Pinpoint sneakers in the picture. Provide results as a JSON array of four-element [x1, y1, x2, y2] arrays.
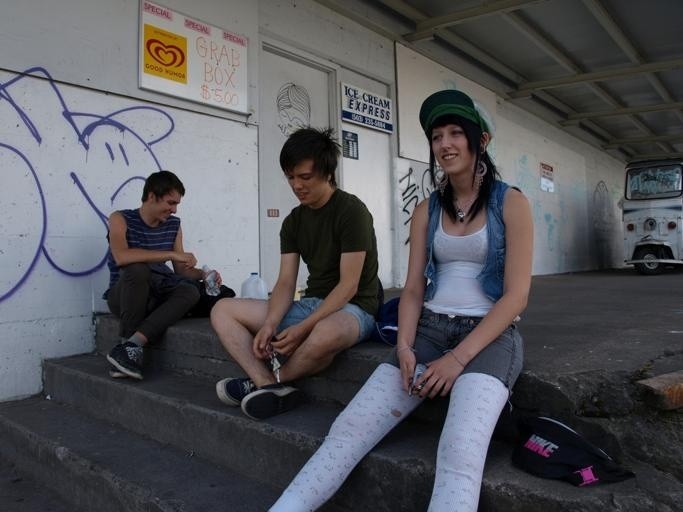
[[106, 342, 145, 380], [239, 383, 303, 422], [215, 376, 259, 408]]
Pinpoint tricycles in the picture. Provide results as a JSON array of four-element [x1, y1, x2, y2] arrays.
[[618, 158, 683, 276]]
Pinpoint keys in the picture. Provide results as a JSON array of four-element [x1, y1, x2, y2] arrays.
[[264, 336, 286, 384]]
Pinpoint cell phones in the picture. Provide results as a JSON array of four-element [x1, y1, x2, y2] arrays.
[[408, 363, 429, 397]]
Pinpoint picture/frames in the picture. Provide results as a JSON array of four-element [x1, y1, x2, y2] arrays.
[[136, 2, 251, 117]]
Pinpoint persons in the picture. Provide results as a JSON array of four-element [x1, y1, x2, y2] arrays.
[[267, 90, 536, 512], [100, 170, 235, 381], [212, 124, 383, 424]]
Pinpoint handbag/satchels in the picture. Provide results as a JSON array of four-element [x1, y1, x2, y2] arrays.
[[511, 413, 638, 487]]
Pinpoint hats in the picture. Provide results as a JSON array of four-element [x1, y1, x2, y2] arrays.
[[371, 294, 400, 347], [418, 88, 495, 140]]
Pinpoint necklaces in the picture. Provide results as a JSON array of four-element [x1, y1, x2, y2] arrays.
[[454, 194, 477, 221]]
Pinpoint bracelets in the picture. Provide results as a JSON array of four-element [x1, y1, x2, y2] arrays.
[[396, 346, 418, 357], [446, 348, 467, 369]]
